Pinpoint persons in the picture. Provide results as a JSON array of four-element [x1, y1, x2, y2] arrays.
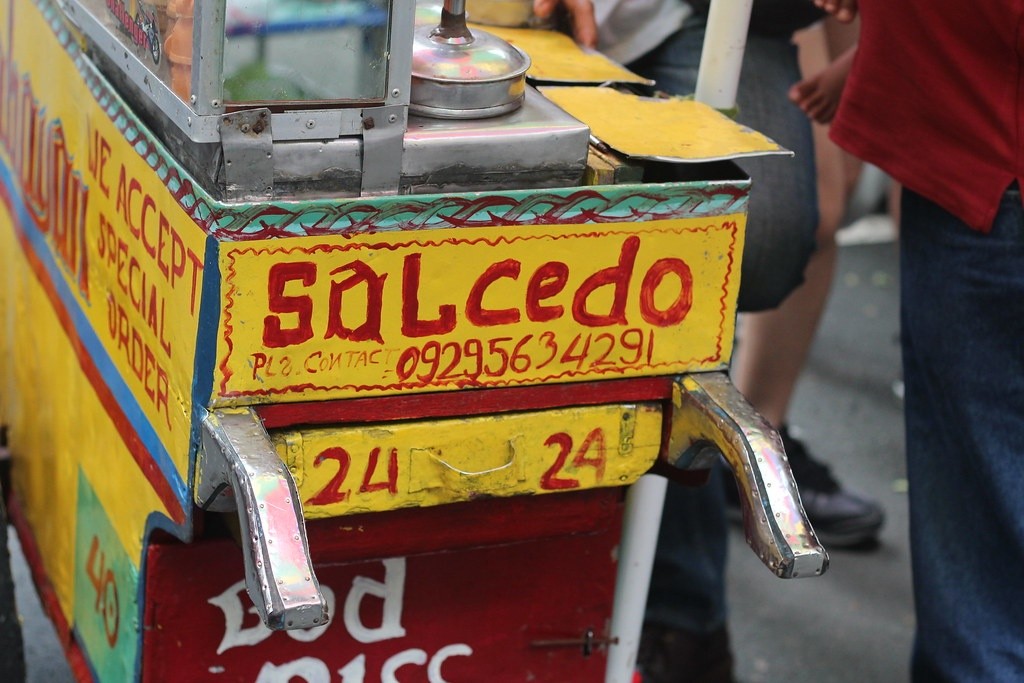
[[786, 0, 856, 123], [832, 0, 1023, 683], [592, 0, 889, 682]]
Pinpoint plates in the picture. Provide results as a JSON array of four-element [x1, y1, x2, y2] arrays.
[[526, 46, 657, 86], [537, 85, 794, 167]]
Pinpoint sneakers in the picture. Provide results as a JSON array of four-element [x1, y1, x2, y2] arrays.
[[724, 425, 887, 546]]
